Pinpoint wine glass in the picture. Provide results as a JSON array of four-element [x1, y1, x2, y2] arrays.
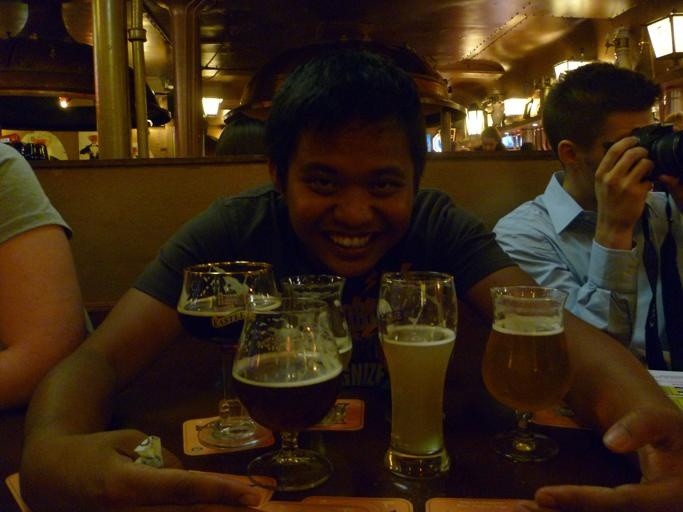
[[484, 284, 567, 463], [231, 297, 343, 494], [176, 259, 283, 451], [287, 275, 353, 452]]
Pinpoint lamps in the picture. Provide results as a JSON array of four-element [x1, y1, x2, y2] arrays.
[[644, 6, 683, 74], [199, 91, 223, 120], [463, 105, 493, 139], [550, 59, 599, 78], [502, 94, 543, 123]]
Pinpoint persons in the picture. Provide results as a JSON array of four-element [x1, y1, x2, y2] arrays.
[[1, 142, 88, 416], [213, 39, 311, 160], [489, 63, 683, 484], [19, 49, 676, 510], [470, 128, 506, 154]]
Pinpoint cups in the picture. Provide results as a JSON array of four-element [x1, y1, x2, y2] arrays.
[[374, 269, 459, 481]]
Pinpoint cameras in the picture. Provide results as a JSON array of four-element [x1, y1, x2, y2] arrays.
[[621, 121, 683, 192]]
